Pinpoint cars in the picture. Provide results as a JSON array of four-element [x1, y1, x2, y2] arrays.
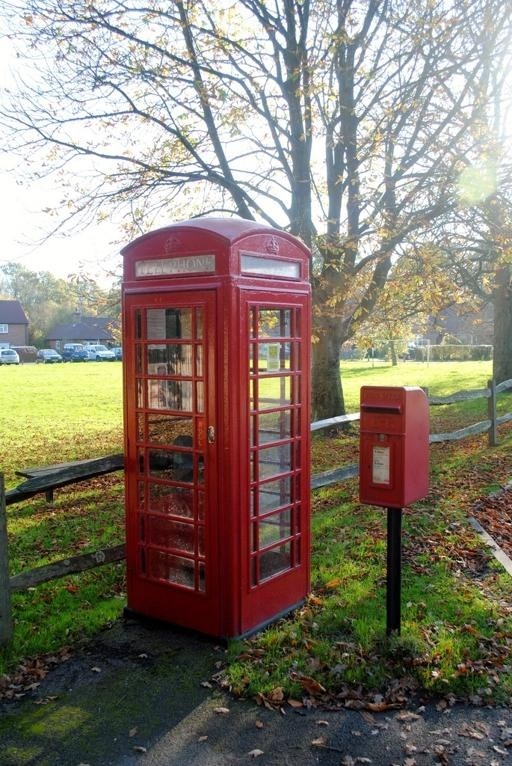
[[111, 347, 123, 360], [1, 349, 20, 366], [36, 350, 62, 363], [263, 344, 290, 359], [63, 351, 86, 362]]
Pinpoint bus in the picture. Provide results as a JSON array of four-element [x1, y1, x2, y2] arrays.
[[64, 343, 84, 352]]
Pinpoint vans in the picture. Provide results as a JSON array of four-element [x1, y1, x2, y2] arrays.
[[12, 345, 38, 361], [87, 345, 116, 361]]
